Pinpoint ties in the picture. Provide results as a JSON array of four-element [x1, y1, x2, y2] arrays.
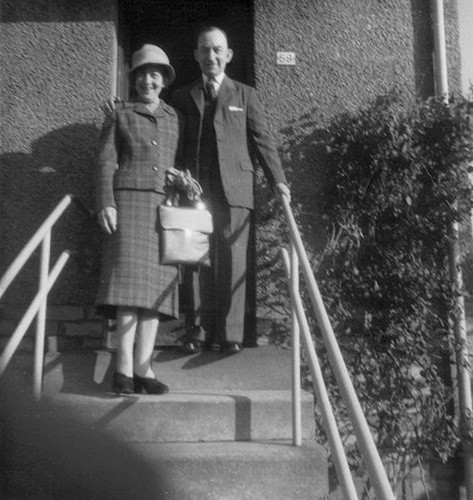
[[204, 79, 217, 100]]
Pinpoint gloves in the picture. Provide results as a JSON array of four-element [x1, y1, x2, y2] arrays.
[[165, 168, 204, 201]]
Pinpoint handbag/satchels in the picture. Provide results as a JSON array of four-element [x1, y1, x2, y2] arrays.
[[156, 186, 214, 268]]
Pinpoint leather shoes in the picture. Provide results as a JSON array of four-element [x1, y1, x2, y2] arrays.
[[221, 342, 240, 354], [184, 338, 207, 355]]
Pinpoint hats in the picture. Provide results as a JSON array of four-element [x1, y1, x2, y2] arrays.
[[125, 44, 177, 89]]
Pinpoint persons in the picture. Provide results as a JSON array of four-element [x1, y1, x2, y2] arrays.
[[98, 24, 292, 355], [93, 44, 204, 395]]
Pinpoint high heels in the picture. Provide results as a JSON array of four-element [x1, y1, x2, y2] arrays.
[[113, 370, 137, 394], [132, 373, 170, 395]]
